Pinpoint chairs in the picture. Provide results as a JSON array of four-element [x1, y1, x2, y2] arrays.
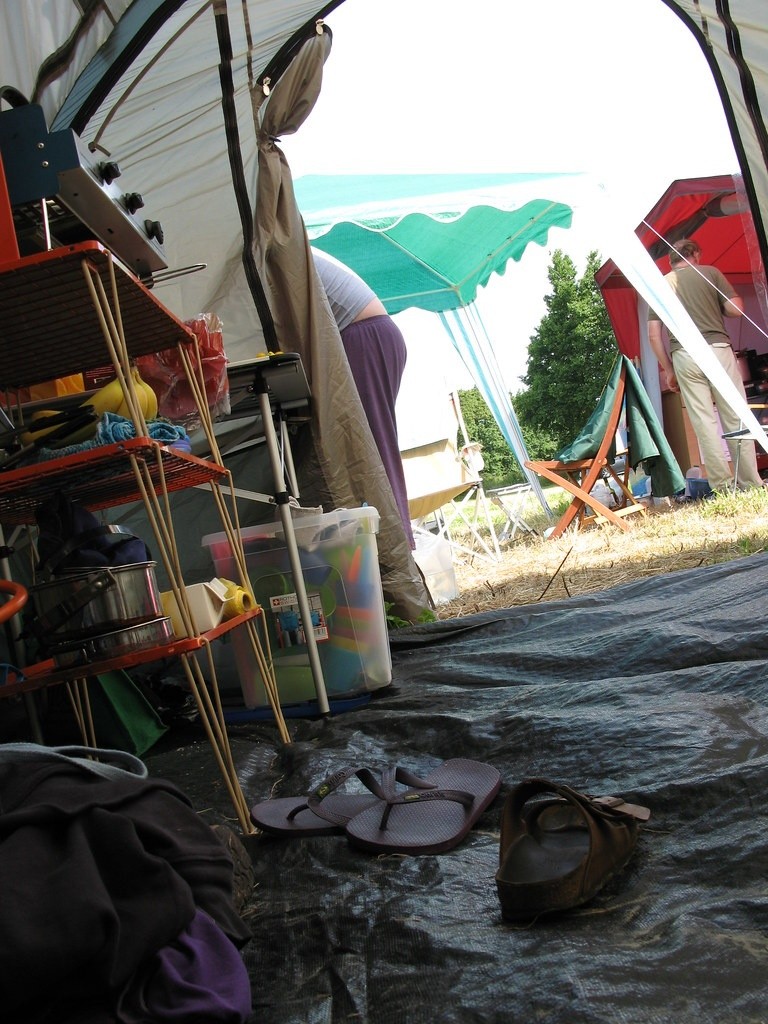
[[722, 403, 768, 497], [525, 355, 649, 541], [399, 439, 498, 561]]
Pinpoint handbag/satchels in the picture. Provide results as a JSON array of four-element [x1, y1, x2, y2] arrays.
[[584, 476, 625, 515]]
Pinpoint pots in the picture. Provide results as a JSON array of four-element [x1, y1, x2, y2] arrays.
[[51, 616, 177, 674], [30, 558, 164, 645]]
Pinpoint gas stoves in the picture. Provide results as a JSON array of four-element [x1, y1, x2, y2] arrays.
[[0, 84, 169, 292]]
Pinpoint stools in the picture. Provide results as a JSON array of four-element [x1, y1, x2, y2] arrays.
[[486, 483, 537, 542]]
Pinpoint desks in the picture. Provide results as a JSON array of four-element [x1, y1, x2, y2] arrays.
[[193, 352, 342, 718]]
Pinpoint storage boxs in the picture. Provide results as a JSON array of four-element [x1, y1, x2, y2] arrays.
[[202, 507, 392, 708]]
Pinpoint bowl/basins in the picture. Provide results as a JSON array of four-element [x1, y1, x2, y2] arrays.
[[688, 477, 710, 499]]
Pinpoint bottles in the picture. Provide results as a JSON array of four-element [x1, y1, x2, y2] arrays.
[[683, 465, 701, 497]]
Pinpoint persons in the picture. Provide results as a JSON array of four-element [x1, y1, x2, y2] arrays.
[[309, 244, 416, 549], [647, 239, 768, 497]]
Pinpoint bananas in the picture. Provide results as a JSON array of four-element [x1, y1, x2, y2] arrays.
[[20, 356, 158, 447]]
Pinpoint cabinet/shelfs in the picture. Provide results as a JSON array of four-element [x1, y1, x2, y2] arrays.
[[0, 152, 291, 833]]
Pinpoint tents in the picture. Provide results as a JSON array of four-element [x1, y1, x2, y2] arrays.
[[283, 172, 581, 520], [593, 173, 768, 358]]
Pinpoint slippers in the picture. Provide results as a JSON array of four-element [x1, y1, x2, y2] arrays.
[[250, 757, 501, 854], [495, 775, 650, 912]]
